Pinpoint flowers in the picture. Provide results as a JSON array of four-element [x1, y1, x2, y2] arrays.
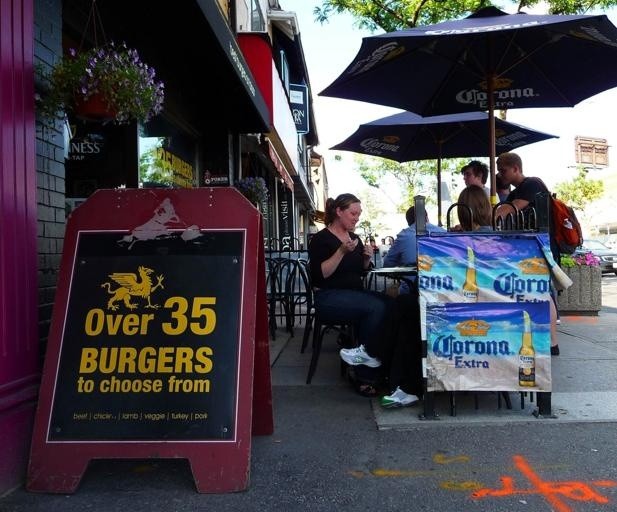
[[234, 177, 270, 202], [42, 41, 166, 124]]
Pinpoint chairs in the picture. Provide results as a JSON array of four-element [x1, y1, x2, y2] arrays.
[[305, 261, 362, 384], [296, 258, 349, 353]]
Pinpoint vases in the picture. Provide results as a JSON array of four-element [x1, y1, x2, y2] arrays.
[[76, 93, 117, 119]]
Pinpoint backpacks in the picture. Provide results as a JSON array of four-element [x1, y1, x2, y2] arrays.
[[549, 194, 584, 254]]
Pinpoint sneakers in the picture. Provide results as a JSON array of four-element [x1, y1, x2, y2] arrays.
[[339, 343, 382, 368], [380, 384, 422, 408]]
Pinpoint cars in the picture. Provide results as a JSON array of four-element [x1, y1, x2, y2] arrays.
[[574, 237, 617, 274]]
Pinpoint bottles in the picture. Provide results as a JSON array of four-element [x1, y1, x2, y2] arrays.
[[462, 262, 479, 302], [518, 326, 535, 386]]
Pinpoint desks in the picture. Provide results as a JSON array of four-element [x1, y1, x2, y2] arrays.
[[367, 267, 418, 340]]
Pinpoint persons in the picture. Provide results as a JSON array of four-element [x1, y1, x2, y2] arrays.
[[311, 195, 420, 408], [457, 153, 498, 228], [382, 205, 446, 296], [494, 152, 560, 356]]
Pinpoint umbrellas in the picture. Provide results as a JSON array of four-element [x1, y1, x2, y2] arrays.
[[318, 5, 617, 232], [329, 110, 559, 227]]
